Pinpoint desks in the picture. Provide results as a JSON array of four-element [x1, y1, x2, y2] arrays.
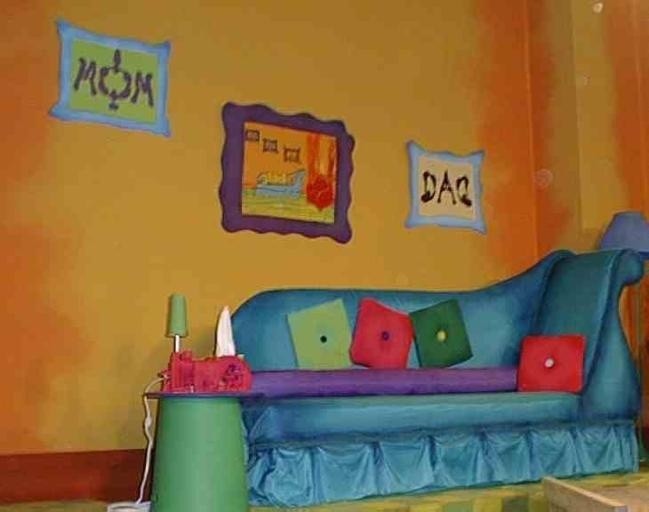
[[144, 391, 259, 512]]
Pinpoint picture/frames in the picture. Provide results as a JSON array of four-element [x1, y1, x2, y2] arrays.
[[47, 16, 172, 138], [218, 102, 354, 244], [405, 141, 486, 235]]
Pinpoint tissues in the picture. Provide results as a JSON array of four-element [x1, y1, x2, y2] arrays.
[[191, 305, 251, 394]]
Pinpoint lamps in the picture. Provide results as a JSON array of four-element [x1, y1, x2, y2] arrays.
[[596, 210, 649, 464], [161, 294, 188, 379]]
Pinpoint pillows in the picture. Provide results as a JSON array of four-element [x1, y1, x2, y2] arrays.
[[517, 334, 585, 394], [349, 300, 413, 368], [409, 300, 474, 368], [287, 300, 354, 372]]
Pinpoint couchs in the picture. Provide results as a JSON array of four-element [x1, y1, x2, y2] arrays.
[[229, 247, 644, 510]]
